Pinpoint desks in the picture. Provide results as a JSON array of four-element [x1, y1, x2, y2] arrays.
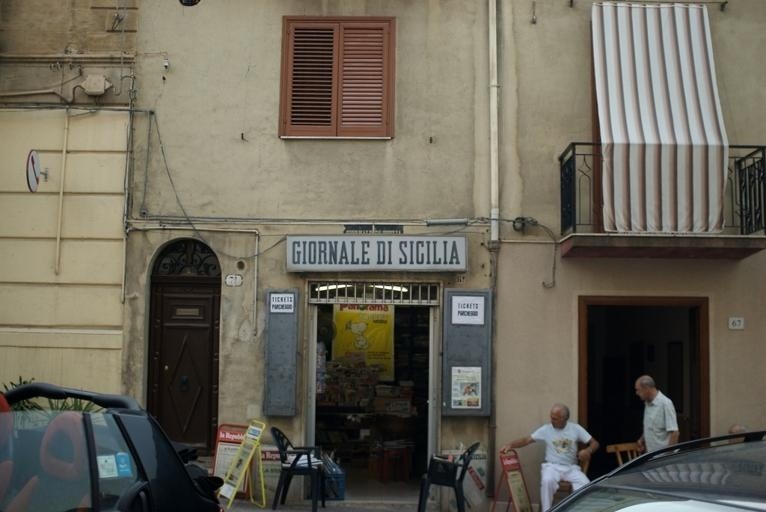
[[316, 404, 424, 480]]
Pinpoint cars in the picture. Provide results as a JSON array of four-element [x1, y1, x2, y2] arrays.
[[544, 430, 765, 511]]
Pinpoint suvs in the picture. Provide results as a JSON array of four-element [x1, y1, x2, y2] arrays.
[[0, 382, 224, 512]]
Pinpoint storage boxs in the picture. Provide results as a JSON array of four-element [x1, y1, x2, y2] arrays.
[[368, 455, 397, 480]]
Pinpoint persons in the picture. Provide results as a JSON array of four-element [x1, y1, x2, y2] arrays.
[[632, 374, 682, 461], [500, 402, 600, 511]]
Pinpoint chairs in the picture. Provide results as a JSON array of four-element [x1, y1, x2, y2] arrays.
[[607, 442, 645, 466], [418, 441, 480, 512], [538, 443, 591, 512], [271, 427, 327, 512]]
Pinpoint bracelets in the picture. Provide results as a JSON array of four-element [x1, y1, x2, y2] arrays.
[[588, 447, 593, 454]]
